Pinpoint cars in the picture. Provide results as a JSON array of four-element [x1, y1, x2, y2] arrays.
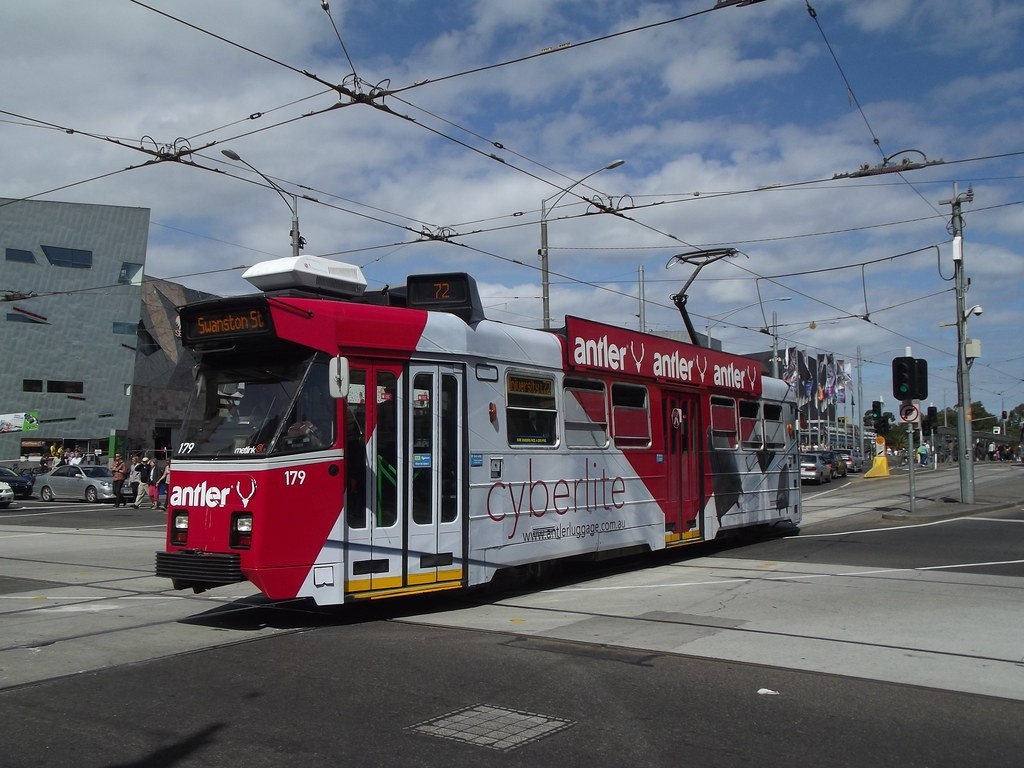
[[805, 451, 847, 480], [832, 449, 863, 473], [31, 464, 134, 504], [0, 481, 14, 509], [0, 465, 33, 500], [799, 453, 832, 485]]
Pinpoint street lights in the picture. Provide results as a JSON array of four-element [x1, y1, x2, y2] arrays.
[[541, 159, 626, 331], [707, 296, 792, 349], [220, 149, 307, 258], [957, 304, 984, 505]]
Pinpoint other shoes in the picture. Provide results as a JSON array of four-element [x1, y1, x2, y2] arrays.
[[152, 505, 158, 510], [131, 504, 138, 509], [113, 504, 119, 508], [124, 499, 128, 505]]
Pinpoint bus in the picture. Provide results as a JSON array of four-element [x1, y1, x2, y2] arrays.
[[156, 247, 803, 609]]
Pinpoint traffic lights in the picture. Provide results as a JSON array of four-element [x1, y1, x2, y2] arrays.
[[872, 401, 880, 420], [874, 417, 886, 436], [1002, 411, 1007, 419], [892, 356, 917, 400]]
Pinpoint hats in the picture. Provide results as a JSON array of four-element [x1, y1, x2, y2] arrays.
[[143, 457, 148, 461]]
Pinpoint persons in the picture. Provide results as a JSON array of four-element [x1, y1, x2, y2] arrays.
[[975, 442, 1013, 462], [918, 440, 930, 467], [225, 382, 290, 417], [111, 452, 128, 508], [40, 442, 85, 467], [894, 447, 908, 462], [13, 463, 22, 475], [886, 446, 892, 456], [128, 456, 170, 511]]
